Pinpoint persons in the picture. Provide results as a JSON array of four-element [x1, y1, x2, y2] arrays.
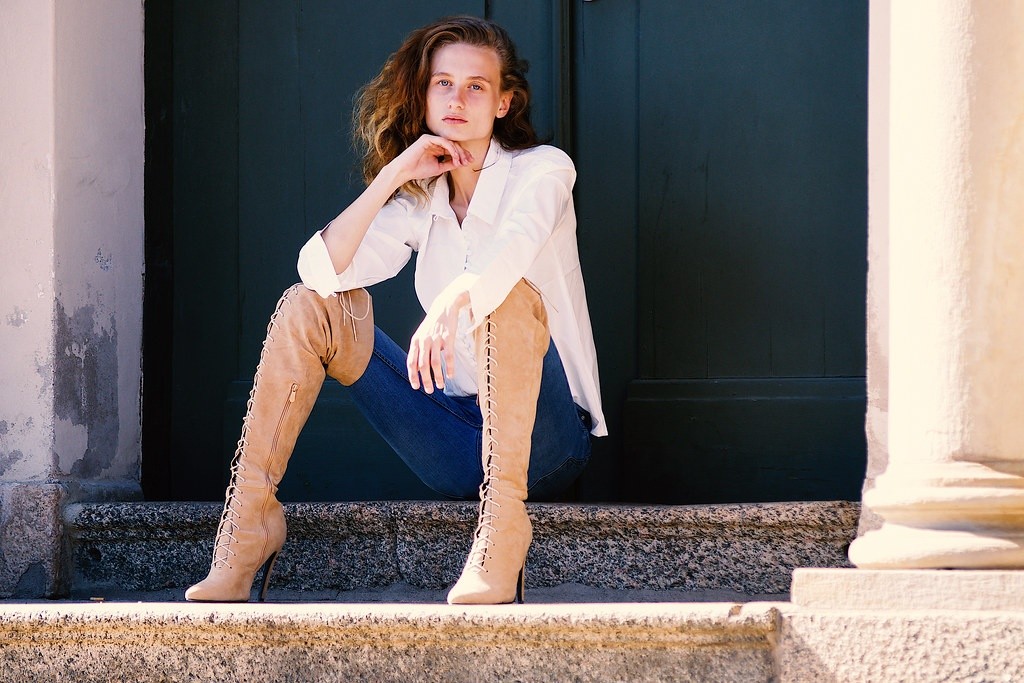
[[183, 13, 606, 605]]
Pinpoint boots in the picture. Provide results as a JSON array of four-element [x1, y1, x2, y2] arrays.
[[186, 283, 377, 602], [448, 278, 552, 606]]
